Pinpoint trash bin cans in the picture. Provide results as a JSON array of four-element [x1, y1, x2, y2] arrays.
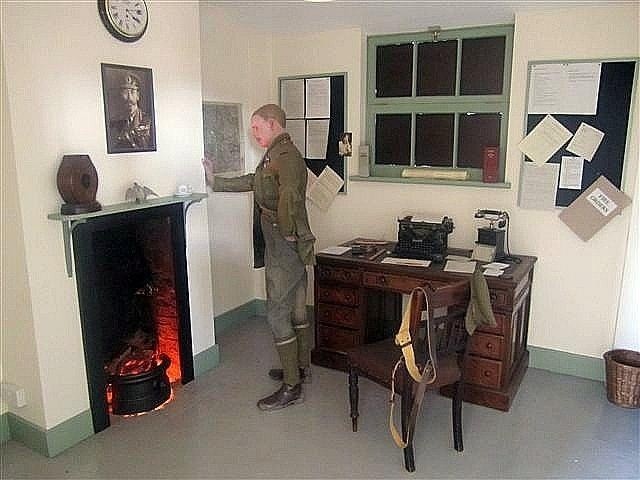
[[603, 349, 640, 409]]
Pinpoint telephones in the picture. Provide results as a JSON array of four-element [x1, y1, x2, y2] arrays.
[[470, 209, 507, 262]]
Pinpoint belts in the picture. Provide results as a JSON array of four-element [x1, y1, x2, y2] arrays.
[[390, 357, 426, 448], [395, 285, 439, 385]]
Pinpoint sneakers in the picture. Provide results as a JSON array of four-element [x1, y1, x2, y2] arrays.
[[258, 384, 304, 410], [269, 366, 312, 384]]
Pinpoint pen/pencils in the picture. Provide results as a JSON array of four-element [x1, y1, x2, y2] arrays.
[[355, 240, 392, 246]]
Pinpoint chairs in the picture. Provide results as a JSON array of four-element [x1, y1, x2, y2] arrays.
[[344, 262, 479, 471]]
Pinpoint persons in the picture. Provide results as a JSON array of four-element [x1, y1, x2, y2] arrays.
[[200, 102, 317, 412], [111, 71, 153, 150]]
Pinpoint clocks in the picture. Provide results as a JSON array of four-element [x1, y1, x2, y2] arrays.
[[96, 0, 149, 43]]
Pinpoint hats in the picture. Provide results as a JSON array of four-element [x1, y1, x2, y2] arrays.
[[120, 71, 142, 90], [465, 270, 497, 336]]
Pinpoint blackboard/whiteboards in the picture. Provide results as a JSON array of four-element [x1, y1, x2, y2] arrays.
[[277, 73, 348, 194], [517, 57, 639, 211]]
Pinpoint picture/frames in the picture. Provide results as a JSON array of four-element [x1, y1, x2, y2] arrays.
[[100, 63, 158, 155]]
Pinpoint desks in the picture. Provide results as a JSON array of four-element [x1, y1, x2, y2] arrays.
[[308, 238, 538, 412]]
